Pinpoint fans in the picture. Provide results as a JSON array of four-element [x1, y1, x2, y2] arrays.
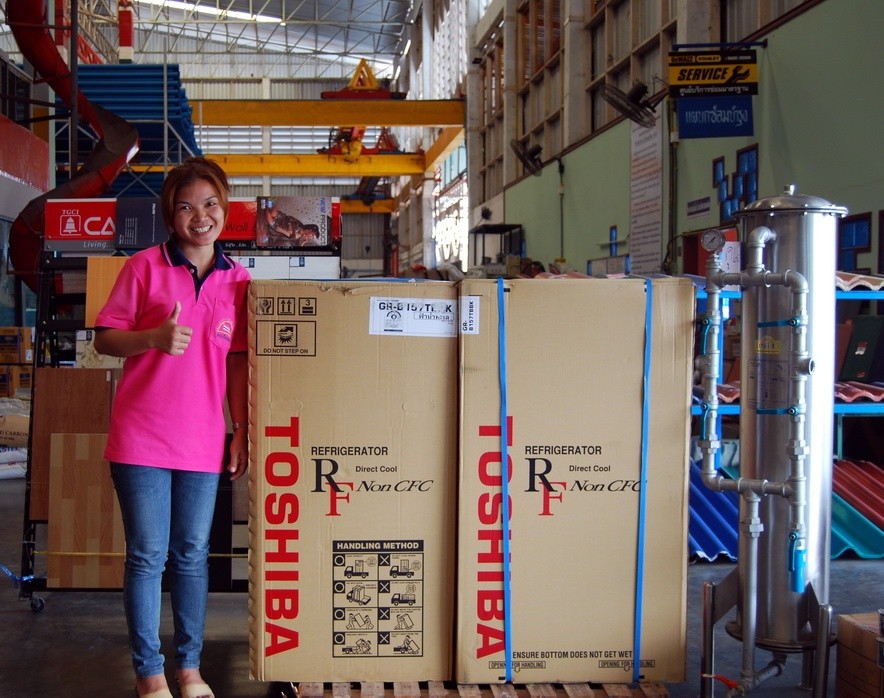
[[511, 139, 543, 176], [598, 79, 671, 130]]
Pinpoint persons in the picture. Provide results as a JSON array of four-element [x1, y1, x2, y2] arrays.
[[257, 197, 320, 247], [92, 156, 252, 694]]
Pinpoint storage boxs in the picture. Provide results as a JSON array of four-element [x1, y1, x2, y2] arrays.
[[455, 280, 696, 686], [245, 279, 460, 684], [0, 327, 36, 447], [834, 612, 884, 698]]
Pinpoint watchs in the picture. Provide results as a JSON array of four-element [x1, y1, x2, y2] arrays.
[[233, 422, 247, 431]]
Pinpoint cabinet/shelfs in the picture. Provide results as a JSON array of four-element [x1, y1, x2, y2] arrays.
[[691, 289, 884, 460]]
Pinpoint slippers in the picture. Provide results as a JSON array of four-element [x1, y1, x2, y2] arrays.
[[134, 679, 173, 698], [174, 674, 215, 698]]
